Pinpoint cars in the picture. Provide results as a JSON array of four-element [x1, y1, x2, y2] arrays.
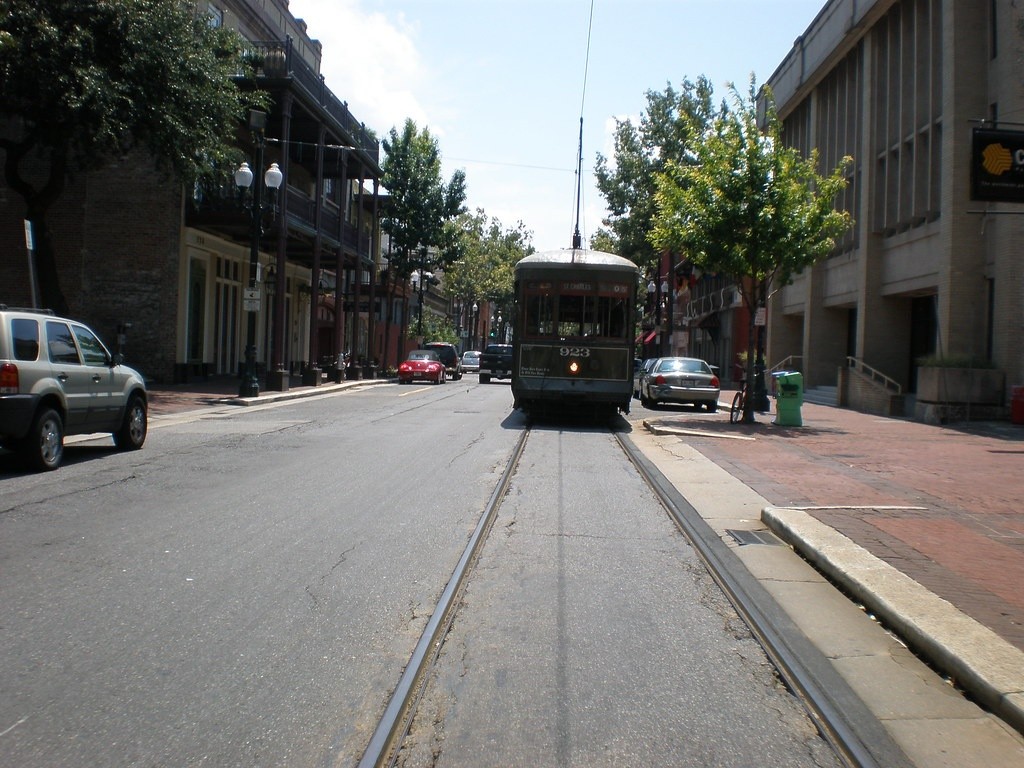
[[632, 358, 659, 401], [458, 350, 483, 374], [398, 350, 446, 386], [640, 356, 720, 413]]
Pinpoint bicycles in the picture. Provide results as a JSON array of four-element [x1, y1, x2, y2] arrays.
[[730, 364, 771, 425]]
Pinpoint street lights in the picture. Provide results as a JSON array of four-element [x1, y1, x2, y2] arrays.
[[411, 269, 434, 349], [468, 302, 478, 350], [235, 127, 283, 397], [647, 280, 656, 358], [490, 315, 503, 344]]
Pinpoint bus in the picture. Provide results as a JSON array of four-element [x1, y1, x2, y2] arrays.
[[509, 247, 643, 418]]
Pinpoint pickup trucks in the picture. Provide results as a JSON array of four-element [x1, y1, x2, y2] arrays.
[[478, 343, 514, 384]]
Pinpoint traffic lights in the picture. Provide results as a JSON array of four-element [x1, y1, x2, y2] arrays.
[[490, 329, 495, 338]]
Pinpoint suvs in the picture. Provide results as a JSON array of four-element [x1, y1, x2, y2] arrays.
[[0, 303, 149, 474], [421, 341, 462, 381]]
